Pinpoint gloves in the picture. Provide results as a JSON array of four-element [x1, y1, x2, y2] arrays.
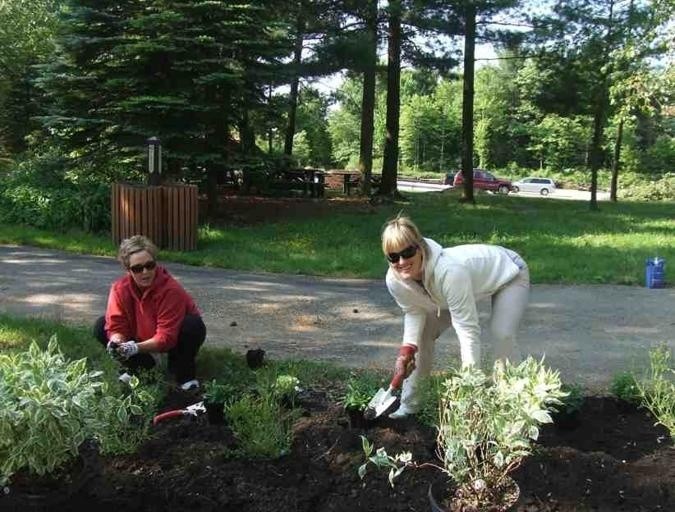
[[393, 343, 419, 378], [105, 338, 138, 368]]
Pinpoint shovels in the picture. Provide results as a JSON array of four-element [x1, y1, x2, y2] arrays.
[[153, 400, 207, 425], [363, 360, 415, 420]]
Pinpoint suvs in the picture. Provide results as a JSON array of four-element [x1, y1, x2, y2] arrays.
[[453, 168, 513, 195]]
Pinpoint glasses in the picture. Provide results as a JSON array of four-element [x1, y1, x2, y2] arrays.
[[126, 260, 156, 273], [386, 245, 419, 263]]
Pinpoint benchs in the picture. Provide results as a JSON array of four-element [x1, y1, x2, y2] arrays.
[[345, 181, 381, 195]]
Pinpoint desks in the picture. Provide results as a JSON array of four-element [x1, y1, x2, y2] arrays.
[[333, 167, 402, 194], [288, 170, 332, 198]]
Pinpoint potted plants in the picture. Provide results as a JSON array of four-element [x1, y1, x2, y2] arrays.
[[357, 351, 572, 512]]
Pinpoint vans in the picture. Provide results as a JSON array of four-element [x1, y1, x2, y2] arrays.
[[511, 176, 557, 197]]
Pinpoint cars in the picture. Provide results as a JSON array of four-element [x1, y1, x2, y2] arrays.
[[444, 169, 459, 186]]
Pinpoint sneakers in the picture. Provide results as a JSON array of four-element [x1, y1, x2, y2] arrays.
[[389, 403, 425, 419], [172, 379, 200, 392], [117, 371, 132, 385]]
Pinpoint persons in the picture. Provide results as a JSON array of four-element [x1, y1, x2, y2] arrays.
[[382, 218, 530, 419], [94, 235, 206, 391]]
